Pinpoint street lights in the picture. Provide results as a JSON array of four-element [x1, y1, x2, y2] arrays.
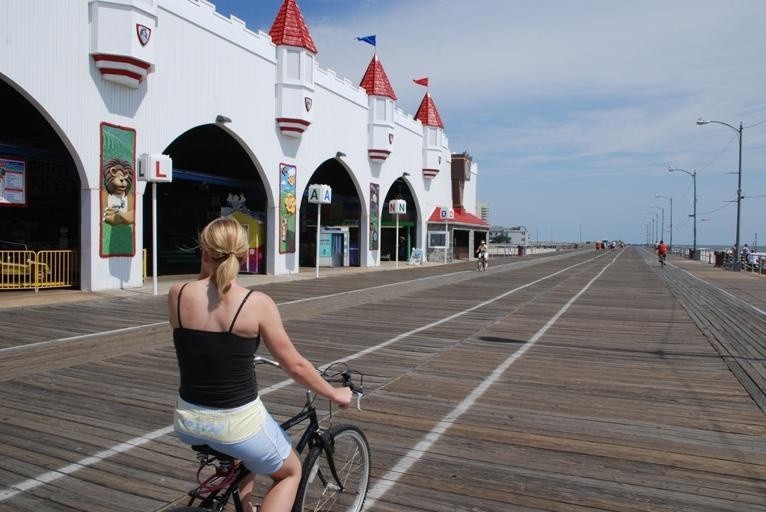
[[644, 193, 672, 252], [668, 166, 698, 260], [696, 118, 743, 271]]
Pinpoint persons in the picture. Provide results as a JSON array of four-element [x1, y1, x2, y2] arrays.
[[733, 244, 737, 267], [658, 240, 667, 264], [166, 215, 353, 512], [475, 240, 488, 269], [748, 249, 758, 268], [743, 244, 750, 263]]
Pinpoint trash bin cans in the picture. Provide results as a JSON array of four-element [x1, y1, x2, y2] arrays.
[[517, 245, 524, 255], [714, 251, 725, 267]]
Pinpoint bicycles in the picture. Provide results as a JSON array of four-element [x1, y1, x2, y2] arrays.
[[659, 255, 666, 268], [476, 250, 488, 271], [166, 353, 372, 510]]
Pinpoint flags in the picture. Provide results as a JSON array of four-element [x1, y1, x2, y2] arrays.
[[356, 35, 376, 47], [414, 77, 428, 87]]
